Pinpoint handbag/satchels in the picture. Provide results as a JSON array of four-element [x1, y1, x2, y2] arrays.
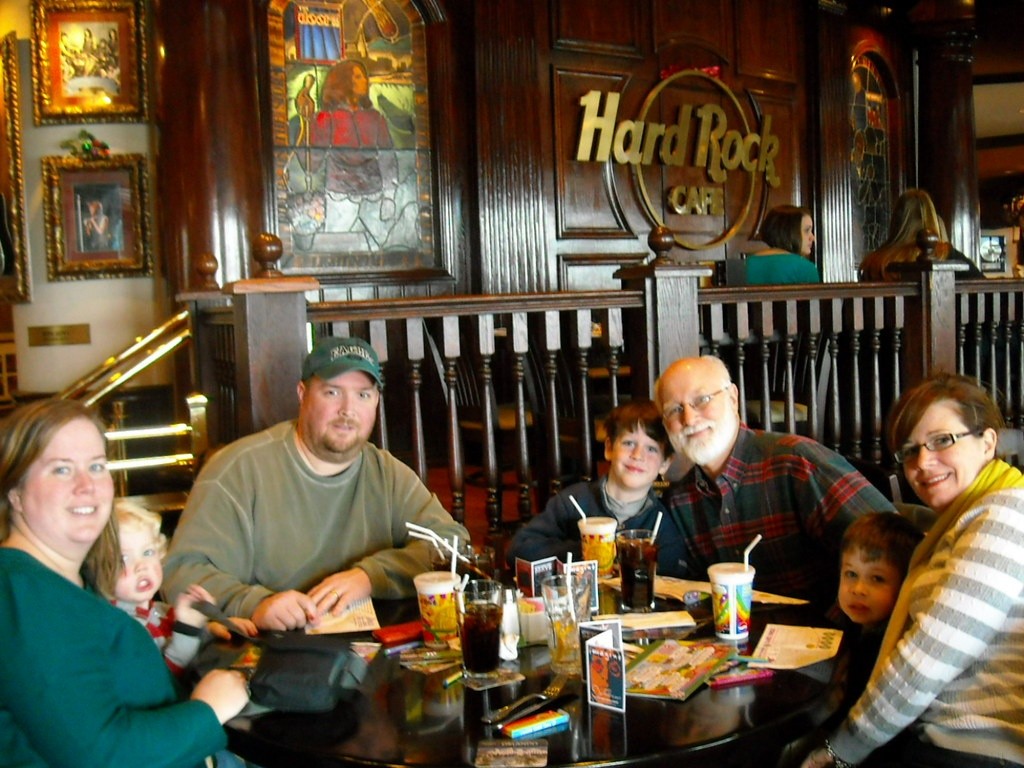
[[170, 601, 365, 711]]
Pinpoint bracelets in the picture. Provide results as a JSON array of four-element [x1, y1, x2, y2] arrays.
[[825, 739, 849, 768]]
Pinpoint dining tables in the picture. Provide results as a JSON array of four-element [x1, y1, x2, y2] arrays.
[[245, 553, 864, 768]]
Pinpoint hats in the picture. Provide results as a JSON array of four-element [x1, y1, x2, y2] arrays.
[[301, 337, 383, 390]]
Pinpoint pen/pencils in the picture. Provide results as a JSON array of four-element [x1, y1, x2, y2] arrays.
[[443, 671, 462, 686], [711, 671, 774, 688], [384, 641, 424, 656]]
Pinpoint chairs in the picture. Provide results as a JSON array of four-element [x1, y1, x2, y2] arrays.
[[560, 312, 648, 425], [498, 313, 637, 478], [420, 312, 535, 491], [745, 324, 827, 437]]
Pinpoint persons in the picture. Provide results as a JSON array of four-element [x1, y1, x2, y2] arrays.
[[0, 398, 265, 768], [505, 398, 686, 575], [160, 337, 473, 634], [857, 188, 986, 282], [798, 371, 1024, 768], [85, 200, 110, 249], [652, 355, 924, 636], [85, 502, 259, 679], [740, 203, 832, 328], [810, 512, 921, 740]]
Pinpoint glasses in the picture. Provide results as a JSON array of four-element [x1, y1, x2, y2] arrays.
[[663, 386, 727, 420], [894, 429, 972, 463]]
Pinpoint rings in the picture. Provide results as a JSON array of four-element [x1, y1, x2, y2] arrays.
[[331, 589, 340, 599]]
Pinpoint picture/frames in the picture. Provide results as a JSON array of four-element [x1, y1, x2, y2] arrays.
[[0, 28, 34, 304], [25, 0, 156, 128], [38, 148, 156, 285]]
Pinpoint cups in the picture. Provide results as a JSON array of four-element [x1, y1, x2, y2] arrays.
[[453, 579, 505, 680], [578, 518, 618, 579], [414, 571, 460, 650], [540, 574, 591, 677], [708, 562, 756, 640], [615, 529, 656, 611]]
